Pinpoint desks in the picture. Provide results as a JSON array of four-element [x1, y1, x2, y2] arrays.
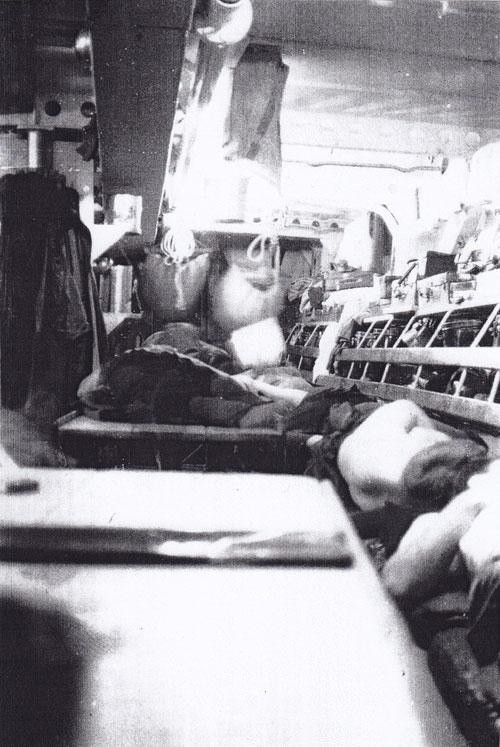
[[0, 468, 468, 747], [51, 411, 308, 473]]
